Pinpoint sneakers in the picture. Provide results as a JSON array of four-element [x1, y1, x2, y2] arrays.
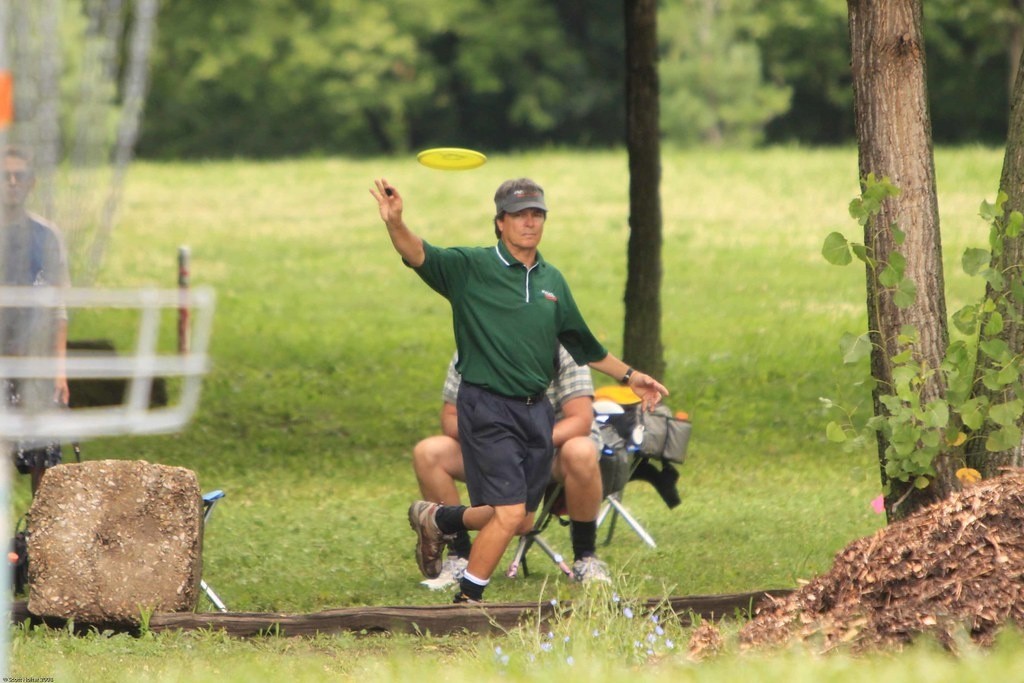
[[572, 556, 612, 587], [419, 557, 469, 590], [452, 592, 481, 604], [408, 500, 457, 579]]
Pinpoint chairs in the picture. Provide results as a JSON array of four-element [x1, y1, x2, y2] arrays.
[[507, 386, 656, 581]]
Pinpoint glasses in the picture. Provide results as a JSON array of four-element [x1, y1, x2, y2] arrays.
[[0, 170, 31, 183]]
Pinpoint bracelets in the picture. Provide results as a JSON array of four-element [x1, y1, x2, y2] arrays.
[[622, 368, 634, 384]]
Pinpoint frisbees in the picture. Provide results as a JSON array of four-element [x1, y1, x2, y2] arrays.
[[417, 147, 487, 171]]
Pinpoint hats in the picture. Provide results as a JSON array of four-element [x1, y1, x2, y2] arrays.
[[494, 177, 549, 215]]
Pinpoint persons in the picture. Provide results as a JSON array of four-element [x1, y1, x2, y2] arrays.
[[414, 342, 610, 592], [0, 145, 69, 474], [369, 177, 669, 604]]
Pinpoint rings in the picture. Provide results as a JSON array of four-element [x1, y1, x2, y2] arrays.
[[385, 189, 392, 196]]
